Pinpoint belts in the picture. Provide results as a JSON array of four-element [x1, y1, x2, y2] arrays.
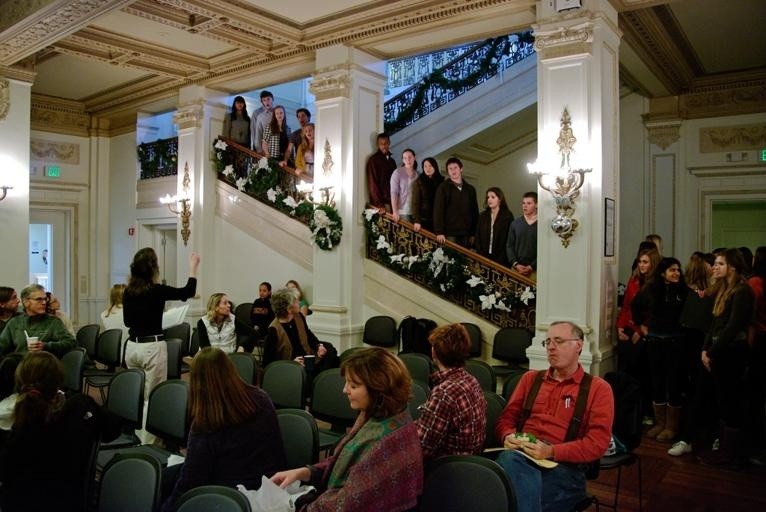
[[129, 336, 164, 343]]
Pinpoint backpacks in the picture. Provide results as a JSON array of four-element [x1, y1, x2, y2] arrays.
[[517, 371, 599, 480]]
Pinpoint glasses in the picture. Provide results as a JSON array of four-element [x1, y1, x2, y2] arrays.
[[541, 338, 578, 347], [25, 297, 48, 303]]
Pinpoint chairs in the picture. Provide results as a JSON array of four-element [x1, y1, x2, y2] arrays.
[[97, 378, 192, 479], [311, 368, 360, 455], [164, 322, 191, 356], [418, 455, 519, 510], [96, 368, 145, 448], [259, 360, 309, 410], [90, 452, 164, 512], [410, 379, 431, 420], [170, 485, 253, 512], [502, 373, 524, 402], [485, 391, 506, 450], [227, 351, 258, 385], [466, 360, 496, 391], [59, 346, 86, 390], [400, 353, 434, 380], [76, 324, 101, 360], [165, 337, 183, 381], [600, 371, 649, 512], [276, 407, 320, 471], [491, 327, 534, 377], [362, 316, 398, 348], [82, 328, 122, 393], [461, 322, 482, 360]]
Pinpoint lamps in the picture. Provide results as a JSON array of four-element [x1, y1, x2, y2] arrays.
[[295, 139, 337, 214], [159, 162, 192, 247], [526, 107, 592, 247]]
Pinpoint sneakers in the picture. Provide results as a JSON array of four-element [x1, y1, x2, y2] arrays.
[[668, 441, 693, 456]]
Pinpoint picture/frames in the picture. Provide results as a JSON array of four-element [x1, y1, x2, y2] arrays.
[[604, 198, 615, 257]]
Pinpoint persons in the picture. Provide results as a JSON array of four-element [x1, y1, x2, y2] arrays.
[[494, 323, 615, 511], [250, 282, 274, 330], [295, 123, 315, 179], [0, 284, 77, 358], [433, 159, 480, 248], [45, 292, 77, 344], [507, 192, 537, 283], [261, 105, 291, 166], [680, 255, 720, 372], [412, 323, 489, 461], [161, 346, 287, 512], [646, 234, 665, 259], [0, 285, 23, 333], [286, 280, 309, 316], [631, 257, 697, 441], [101, 284, 130, 363], [390, 150, 422, 225], [616, 250, 658, 427], [411, 158, 442, 232], [669, 249, 757, 462], [278, 108, 311, 170], [0, 355, 66, 430], [742, 246, 766, 467], [124, 247, 200, 444], [262, 290, 327, 371], [222, 96, 250, 149], [0, 351, 122, 512], [270, 348, 423, 512], [366, 132, 396, 216], [194, 293, 237, 353], [469, 187, 514, 267], [254, 90, 273, 154], [741, 247, 753, 275]]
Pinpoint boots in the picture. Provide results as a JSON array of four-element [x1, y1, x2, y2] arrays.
[[656, 405, 681, 442], [646, 402, 666, 439]]
